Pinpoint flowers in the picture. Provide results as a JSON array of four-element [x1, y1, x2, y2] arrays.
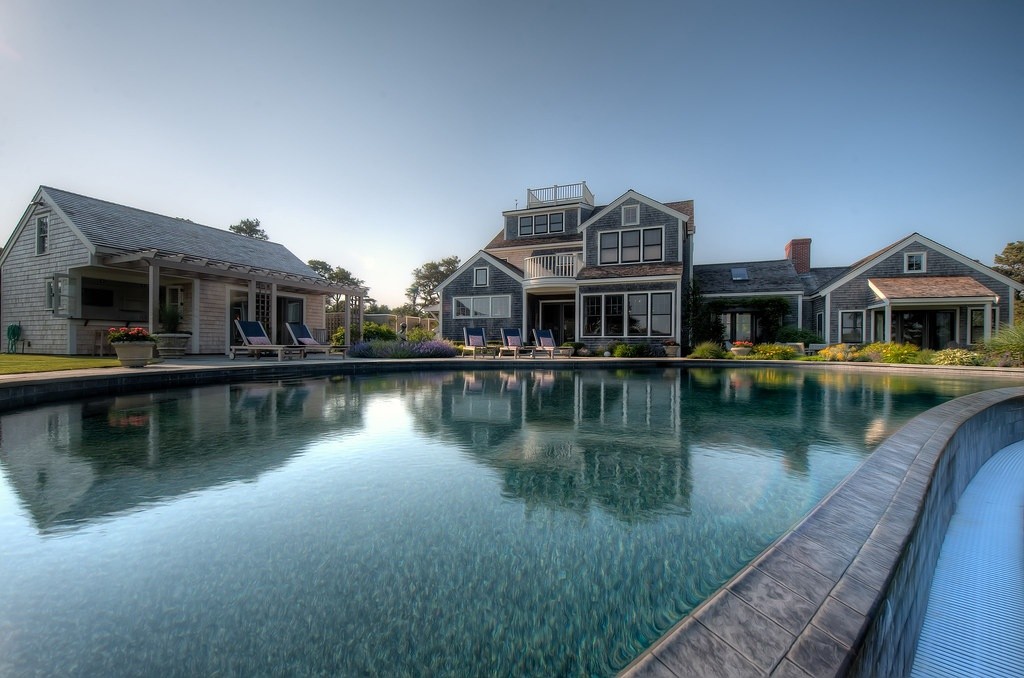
[[661, 338, 679, 346], [733, 340, 753, 348], [106, 327, 155, 342]]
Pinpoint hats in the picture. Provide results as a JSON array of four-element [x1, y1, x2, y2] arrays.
[[400, 322, 407, 327]]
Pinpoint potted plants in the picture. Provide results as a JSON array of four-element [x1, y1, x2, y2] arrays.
[[577, 347, 591, 356], [559, 342, 575, 357], [153, 306, 193, 359]]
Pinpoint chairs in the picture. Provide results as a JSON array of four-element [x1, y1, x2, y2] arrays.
[[462, 327, 497, 360], [531, 329, 573, 359], [284, 322, 351, 360], [498, 327, 536, 359], [230, 320, 306, 361]]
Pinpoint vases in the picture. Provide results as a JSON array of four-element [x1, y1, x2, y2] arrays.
[[730, 347, 752, 356], [663, 346, 680, 357], [110, 341, 156, 367]]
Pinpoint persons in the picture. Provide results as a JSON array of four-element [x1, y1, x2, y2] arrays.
[[398, 322, 407, 342]]
